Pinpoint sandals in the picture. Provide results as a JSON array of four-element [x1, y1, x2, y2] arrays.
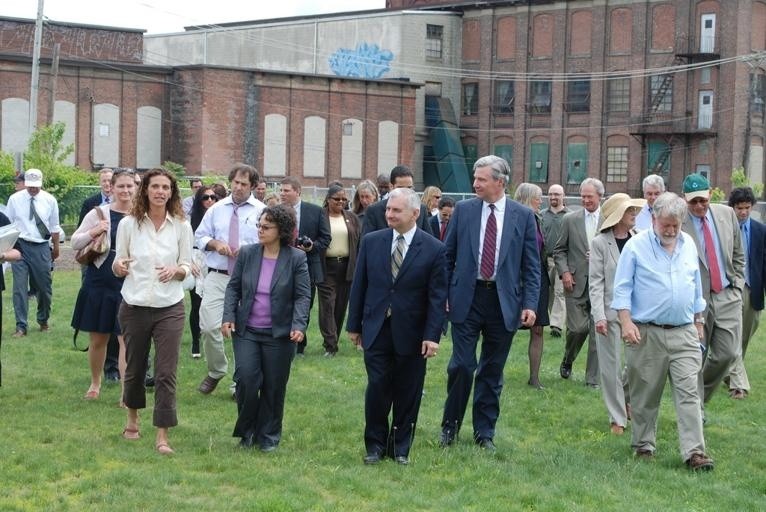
[[154, 444, 175, 454], [84, 392, 100, 400], [105, 375, 118, 384], [191, 340, 201, 359], [121, 428, 141, 442]]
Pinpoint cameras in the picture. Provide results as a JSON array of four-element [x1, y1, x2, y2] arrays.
[[295, 235, 312, 248]]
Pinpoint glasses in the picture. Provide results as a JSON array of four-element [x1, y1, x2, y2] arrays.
[[113, 168, 135, 174], [330, 197, 348, 201], [432, 196, 442, 199], [201, 195, 217, 200]]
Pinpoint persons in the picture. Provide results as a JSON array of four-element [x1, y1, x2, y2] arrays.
[[589, 192, 647, 437], [77, 169, 114, 279], [682, 173, 746, 403], [189, 187, 217, 359], [323, 181, 349, 209], [194, 165, 268, 395], [609, 191, 715, 472], [318, 185, 359, 359], [422, 185, 441, 215], [539, 184, 567, 338], [359, 166, 433, 238], [12, 174, 65, 298], [729, 187, 766, 361], [71, 169, 142, 408], [634, 173, 665, 236], [555, 178, 606, 389], [278, 176, 332, 358], [345, 188, 448, 466], [112, 169, 195, 455], [222, 204, 312, 453], [252, 177, 267, 202], [208, 184, 226, 201], [182, 178, 202, 214], [514, 184, 549, 391], [439, 156, 541, 453], [6, 169, 65, 337], [263, 192, 280, 209], [429, 196, 455, 241], [0, 213, 24, 387], [350, 179, 380, 236]]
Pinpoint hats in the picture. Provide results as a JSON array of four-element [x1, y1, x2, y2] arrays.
[[24, 168, 43, 187], [11, 174, 26, 181], [681, 173, 710, 200], [599, 192, 648, 233]]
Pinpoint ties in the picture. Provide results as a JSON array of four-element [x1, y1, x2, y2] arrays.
[[700, 216, 721, 293], [227, 205, 239, 274], [480, 203, 498, 280], [385, 233, 403, 317], [440, 220, 447, 240], [740, 222, 751, 287], [28, 197, 52, 240], [292, 208, 298, 249]]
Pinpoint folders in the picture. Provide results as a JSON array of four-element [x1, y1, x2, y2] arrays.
[[0, 222, 17, 236]]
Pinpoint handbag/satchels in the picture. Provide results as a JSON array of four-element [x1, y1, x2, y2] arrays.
[[75, 206, 107, 262]]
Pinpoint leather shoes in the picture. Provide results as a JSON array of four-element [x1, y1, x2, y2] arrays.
[[26, 291, 36, 298], [625, 402, 632, 419], [240, 431, 255, 448], [632, 448, 656, 466], [40, 323, 49, 332], [438, 432, 455, 447], [144, 375, 156, 387], [610, 421, 624, 434], [584, 382, 599, 388], [297, 353, 306, 358], [527, 379, 548, 390], [11, 330, 27, 338], [549, 326, 561, 336], [321, 351, 338, 358], [258, 434, 277, 453], [731, 387, 746, 400], [391, 454, 410, 465], [362, 454, 382, 465], [199, 375, 222, 393], [477, 437, 498, 451], [561, 361, 573, 378], [686, 453, 714, 472]]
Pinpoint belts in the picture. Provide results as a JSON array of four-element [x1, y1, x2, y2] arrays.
[[474, 282, 499, 289], [638, 322, 695, 330], [208, 267, 228, 275], [324, 255, 351, 263]]
